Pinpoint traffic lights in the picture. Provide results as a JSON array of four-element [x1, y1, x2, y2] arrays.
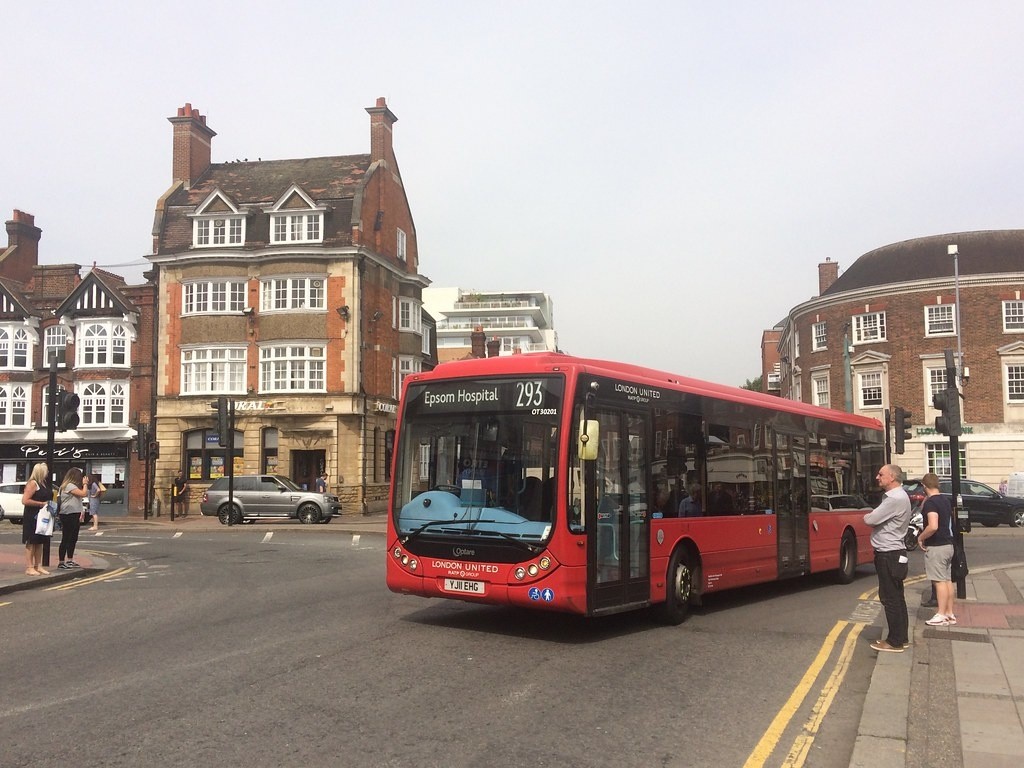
[[894, 408, 913, 454], [210, 397, 229, 448], [149, 442, 158, 460], [931, 387, 961, 437], [57, 392, 79, 432]]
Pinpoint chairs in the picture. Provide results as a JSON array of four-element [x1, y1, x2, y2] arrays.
[[598, 493, 644, 521]]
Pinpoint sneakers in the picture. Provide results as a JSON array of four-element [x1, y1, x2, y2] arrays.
[[925, 613, 957, 626], [57, 564, 73, 570], [66, 561, 80, 567]]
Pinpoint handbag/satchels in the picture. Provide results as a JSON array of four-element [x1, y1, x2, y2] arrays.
[[34, 503, 55, 536], [98, 481, 107, 495]]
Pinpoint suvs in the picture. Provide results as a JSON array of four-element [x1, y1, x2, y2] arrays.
[[902, 476, 1024, 529], [200, 474, 341, 527], [0, 481, 93, 527]]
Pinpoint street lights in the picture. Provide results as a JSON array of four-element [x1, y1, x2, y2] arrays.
[[947, 243, 970, 424]]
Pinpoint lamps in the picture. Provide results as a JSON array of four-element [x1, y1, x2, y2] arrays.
[[241, 306, 254, 315], [336, 305, 349, 320], [369, 311, 383, 322]]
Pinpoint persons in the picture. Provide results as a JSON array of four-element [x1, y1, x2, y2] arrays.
[[999, 479, 1008, 496], [917, 473, 956, 626], [87, 474, 107, 531], [311, 473, 328, 492], [662, 479, 750, 516], [457, 442, 503, 496], [57, 467, 89, 571], [863, 465, 912, 653], [21, 462, 54, 577], [173, 470, 187, 519]]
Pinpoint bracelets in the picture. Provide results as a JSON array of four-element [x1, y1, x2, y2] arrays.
[[83, 483, 88, 485]]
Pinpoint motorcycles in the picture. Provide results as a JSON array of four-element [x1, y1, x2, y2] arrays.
[[904, 503, 928, 552]]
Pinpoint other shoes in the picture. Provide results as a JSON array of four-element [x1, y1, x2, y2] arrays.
[[921, 599, 938, 607], [870, 640, 909, 653], [25, 567, 40, 576], [34, 565, 50, 575]]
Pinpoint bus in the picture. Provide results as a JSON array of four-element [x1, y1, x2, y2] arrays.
[[384, 351, 891, 627]]
